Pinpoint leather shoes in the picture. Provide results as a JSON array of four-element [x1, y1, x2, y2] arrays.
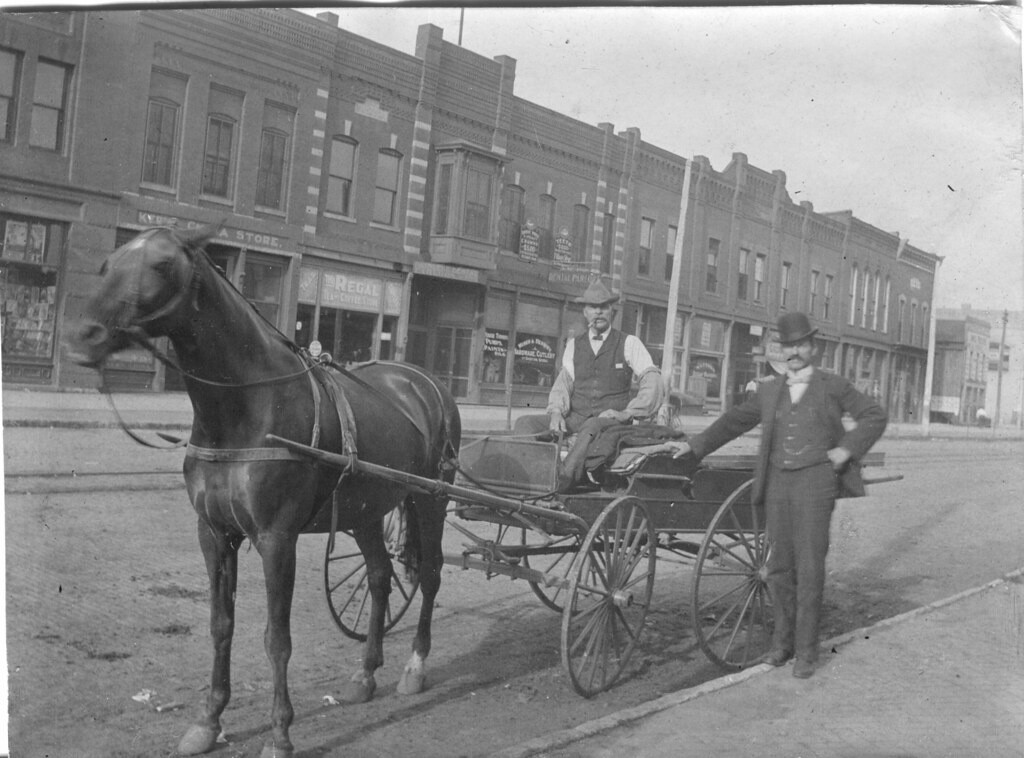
[[792, 652, 815, 678], [762, 646, 794, 666]]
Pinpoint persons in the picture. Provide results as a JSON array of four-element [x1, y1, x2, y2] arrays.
[[665, 313, 889, 682], [512, 281, 664, 499]]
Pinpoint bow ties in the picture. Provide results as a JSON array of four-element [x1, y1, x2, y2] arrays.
[[785, 376, 812, 386]]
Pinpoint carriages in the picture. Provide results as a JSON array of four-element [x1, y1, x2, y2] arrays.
[[61, 218, 774, 758]]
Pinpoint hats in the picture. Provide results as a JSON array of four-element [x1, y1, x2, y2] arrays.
[[771, 312, 819, 345], [574, 280, 620, 306]]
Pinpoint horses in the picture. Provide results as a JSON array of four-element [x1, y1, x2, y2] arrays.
[[63, 216, 462, 758]]
